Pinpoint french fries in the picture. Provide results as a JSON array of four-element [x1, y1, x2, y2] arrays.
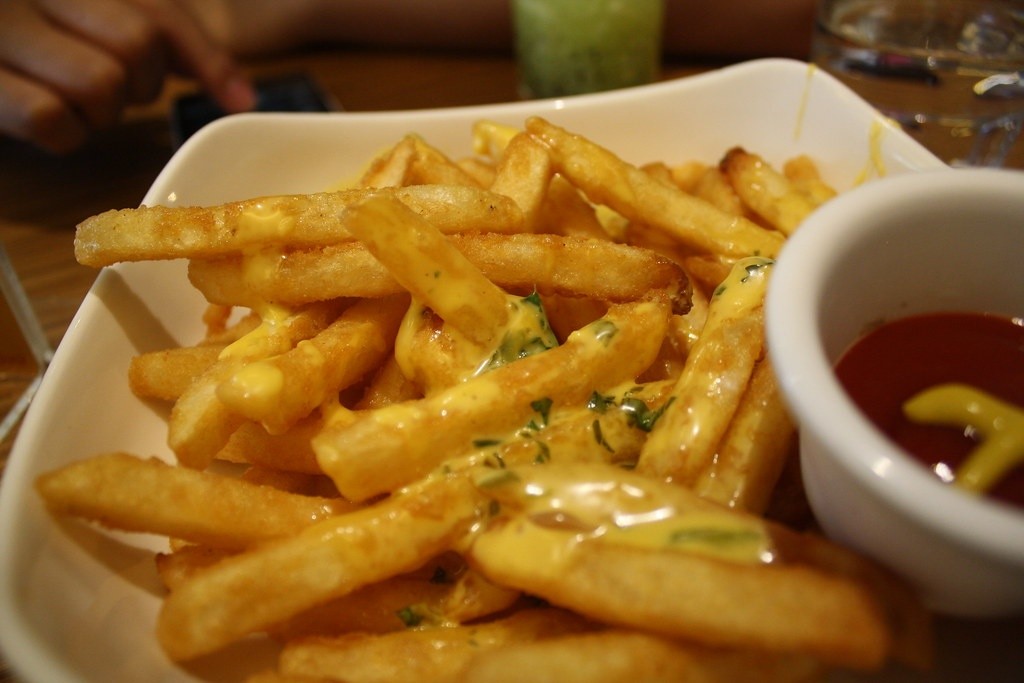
[[32, 119, 935, 683]]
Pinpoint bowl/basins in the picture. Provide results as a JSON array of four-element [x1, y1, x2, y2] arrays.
[[763, 166, 1024, 611]]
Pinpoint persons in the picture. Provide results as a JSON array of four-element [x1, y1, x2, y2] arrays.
[[0, 0, 814, 151]]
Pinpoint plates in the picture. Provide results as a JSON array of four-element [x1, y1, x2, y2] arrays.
[[1, 60, 1024, 683]]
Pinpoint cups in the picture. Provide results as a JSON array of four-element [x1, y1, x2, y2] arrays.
[[514, 0, 663, 101], [815, 1, 1024, 169]]
